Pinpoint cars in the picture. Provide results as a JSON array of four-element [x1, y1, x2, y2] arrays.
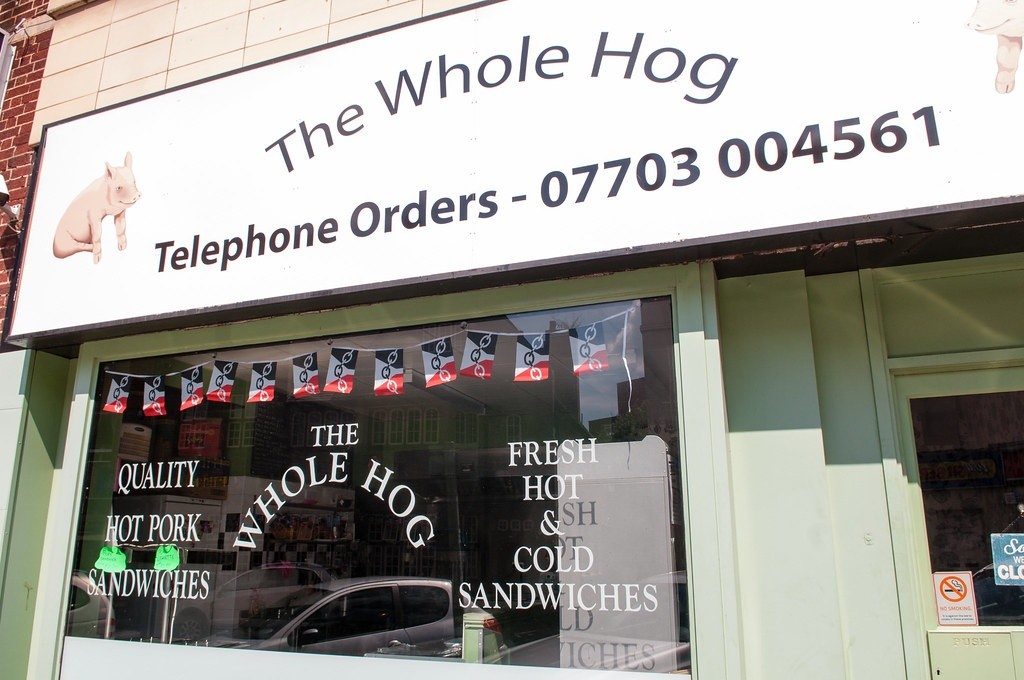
[[67, 569, 116, 640], [178, 573, 512, 666], [612, 570, 690, 643], [170, 559, 344, 645]]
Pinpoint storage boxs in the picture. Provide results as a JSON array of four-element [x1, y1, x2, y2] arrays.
[[112, 495, 222, 549]]
